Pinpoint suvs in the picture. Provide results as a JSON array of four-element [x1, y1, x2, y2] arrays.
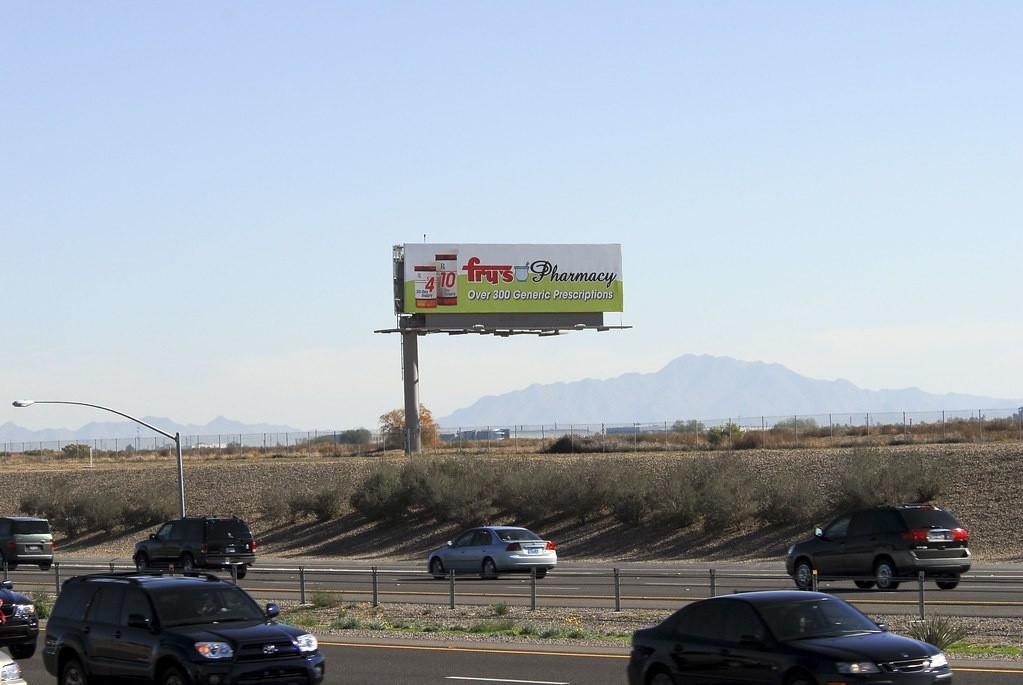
[[42, 572, 325, 685], [784, 503, 972, 593], [132, 515, 257, 579]]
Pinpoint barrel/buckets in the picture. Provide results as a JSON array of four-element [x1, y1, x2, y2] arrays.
[[434, 253, 457, 305], [415, 265, 436, 308]]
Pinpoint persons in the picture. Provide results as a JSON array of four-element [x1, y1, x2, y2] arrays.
[[188, 589, 231, 618]]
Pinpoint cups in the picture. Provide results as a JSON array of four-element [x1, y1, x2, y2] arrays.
[[514, 266, 529, 282]]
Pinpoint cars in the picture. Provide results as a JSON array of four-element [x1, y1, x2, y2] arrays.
[[0, 580, 39, 660], [427, 526, 560, 582], [0, 516, 54, 573], [626, 591, 954, 685]]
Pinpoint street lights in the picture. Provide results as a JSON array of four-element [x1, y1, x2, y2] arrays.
[[12, 399, 186, 519]]
